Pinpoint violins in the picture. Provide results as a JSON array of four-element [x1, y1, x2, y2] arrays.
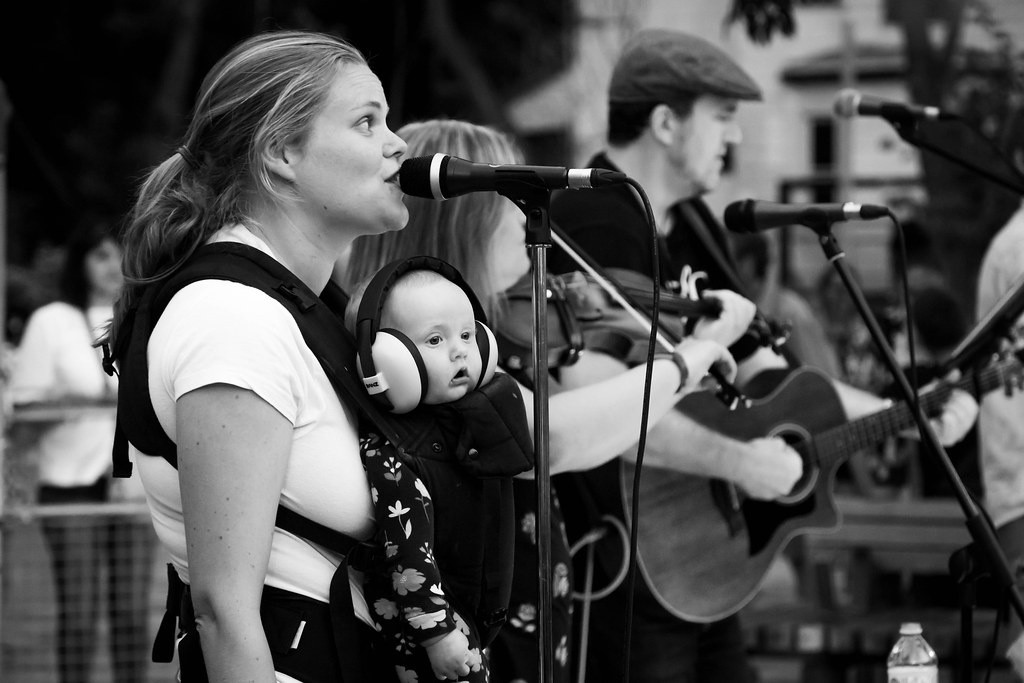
[[486, 271, 793, 366]]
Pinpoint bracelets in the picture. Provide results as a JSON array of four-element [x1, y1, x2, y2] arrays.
[[653, 353, 690, 395]]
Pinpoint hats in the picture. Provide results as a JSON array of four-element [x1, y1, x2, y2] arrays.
[[611, 29, 761, 102]]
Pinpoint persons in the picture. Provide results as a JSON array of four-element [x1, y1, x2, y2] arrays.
[[537, 28, 1021, 683], [97, 29, 408, 683], [2, 235, 155, 683], [340, 121, 755, 486], [344, 253, 532, 683]]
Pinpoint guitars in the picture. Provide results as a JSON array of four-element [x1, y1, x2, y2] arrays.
[[597, 346, 1023, 632]]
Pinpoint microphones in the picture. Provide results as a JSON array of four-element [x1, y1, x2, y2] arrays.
[[399, 153, 626, 202], [832, 89, 956, 122], [724, 199, 889, 232]]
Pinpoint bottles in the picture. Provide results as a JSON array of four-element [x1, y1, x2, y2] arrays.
[[887, 623, 939, 683]]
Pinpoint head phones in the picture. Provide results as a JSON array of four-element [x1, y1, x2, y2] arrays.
[[352, 254, 499, 416]]
